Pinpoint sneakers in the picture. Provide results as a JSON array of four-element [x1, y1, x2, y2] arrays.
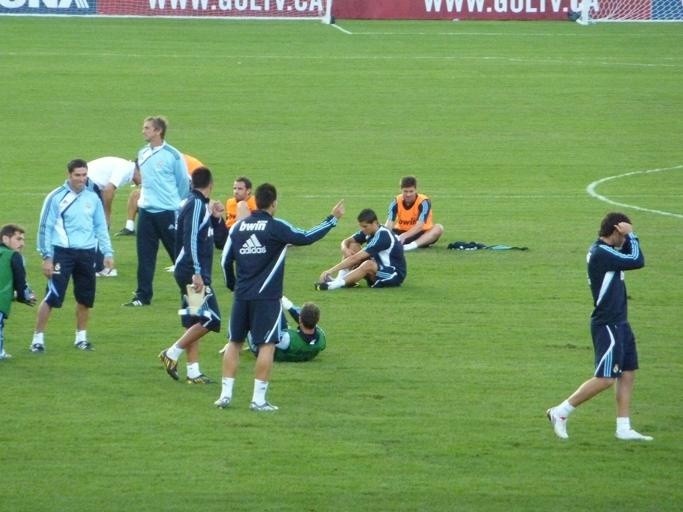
[[249, 401, 279, 413], [157, 348, 180, 381], [312, 282, 329, 293], [74, 341, 95, 353], [29, 343, 47, 355], [546, 407, 570, 440], [113, 228, 136, 237], [185, 373, 216, 385], [614, 428, 654, 441], [95, 268, 118, 277], [214, 396, 231, 409], [121, 297, 144, 308]]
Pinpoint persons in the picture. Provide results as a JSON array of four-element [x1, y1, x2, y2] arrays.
[[219, 296, 326, 363], [213, 177, 256, 250], [0, 224, 36, 360], [85, 157, 141, 277], [115, 153, 205, 235], [547, 213, 653, 441], [214, 184, 345, 411], [314, 209, 407, 291], [158, 166, 224, 385], [30, 159, 113, 352], [385, 177, 444, 251], [122, 117, 189, 306]]
